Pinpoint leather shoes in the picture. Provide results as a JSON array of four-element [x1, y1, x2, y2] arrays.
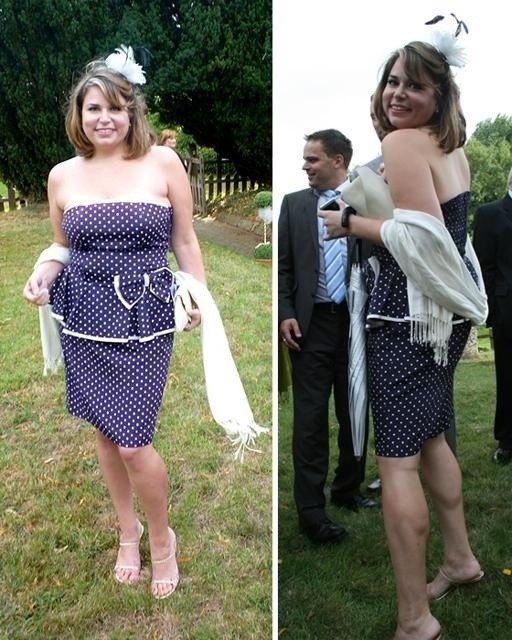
[[491, 440, 512, 466], [329, 494, 378, 513], [366, 478, 381, 492], [298, 516, 347, 546]]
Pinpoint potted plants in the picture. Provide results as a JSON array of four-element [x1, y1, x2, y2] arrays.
[[253, 191, 272, 267]]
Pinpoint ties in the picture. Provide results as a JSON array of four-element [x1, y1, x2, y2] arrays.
[[323, 189, 346, 305]]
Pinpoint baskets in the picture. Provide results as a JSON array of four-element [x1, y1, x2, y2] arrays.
[[173, 285, 192, 334]]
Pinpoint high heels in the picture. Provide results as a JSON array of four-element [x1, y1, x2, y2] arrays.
[[150, 526, 180, 600], [112, 518, 144, 585]]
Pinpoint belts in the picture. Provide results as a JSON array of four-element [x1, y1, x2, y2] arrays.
[[312, 301, 349, 314]]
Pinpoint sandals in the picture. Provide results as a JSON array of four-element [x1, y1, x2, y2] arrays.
[[436, 634, 442, 640], [435, 568, 484, 601]]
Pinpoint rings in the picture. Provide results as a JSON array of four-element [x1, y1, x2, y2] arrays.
[[283, 338, 287, 342]]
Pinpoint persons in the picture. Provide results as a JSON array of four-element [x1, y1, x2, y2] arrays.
[[279, 126, 380, 545], [21, 58, 209, 600], [158, 128, 188, 173], [317, 40, 490, 639], [472, 168, 512, 462]]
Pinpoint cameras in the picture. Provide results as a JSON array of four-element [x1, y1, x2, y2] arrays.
[[319, 197, 340, 211]]
